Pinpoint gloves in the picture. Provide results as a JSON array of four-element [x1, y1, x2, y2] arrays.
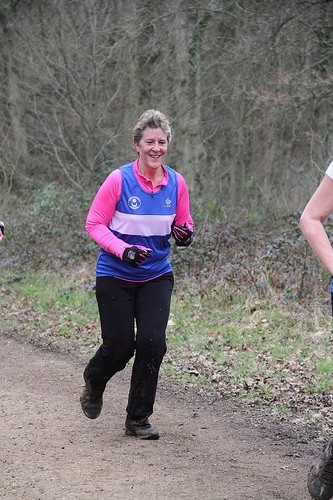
[[171, 222, 192, 247], [121, 245, 152, 268]]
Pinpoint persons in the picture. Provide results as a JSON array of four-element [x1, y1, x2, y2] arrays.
[[79, 109, 196, 441], [298, 157, 332, 500]]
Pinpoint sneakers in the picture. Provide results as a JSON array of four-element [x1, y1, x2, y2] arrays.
[[124, 417, 159, 439], [306, 438, 332, 500], [79, 371, 102, 419]]
